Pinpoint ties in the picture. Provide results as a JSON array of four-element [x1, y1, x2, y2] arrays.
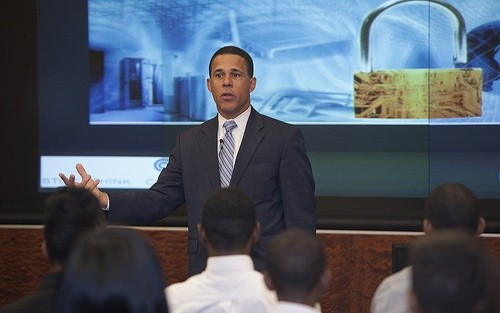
[[218, 121, 237, 189]]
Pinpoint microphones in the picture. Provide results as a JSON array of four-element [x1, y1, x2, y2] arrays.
[[220, 139, 223, 144]]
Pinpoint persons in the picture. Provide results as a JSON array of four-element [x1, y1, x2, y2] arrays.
[[59, 46, 317, 277], [0, 184, 500, 313]]
